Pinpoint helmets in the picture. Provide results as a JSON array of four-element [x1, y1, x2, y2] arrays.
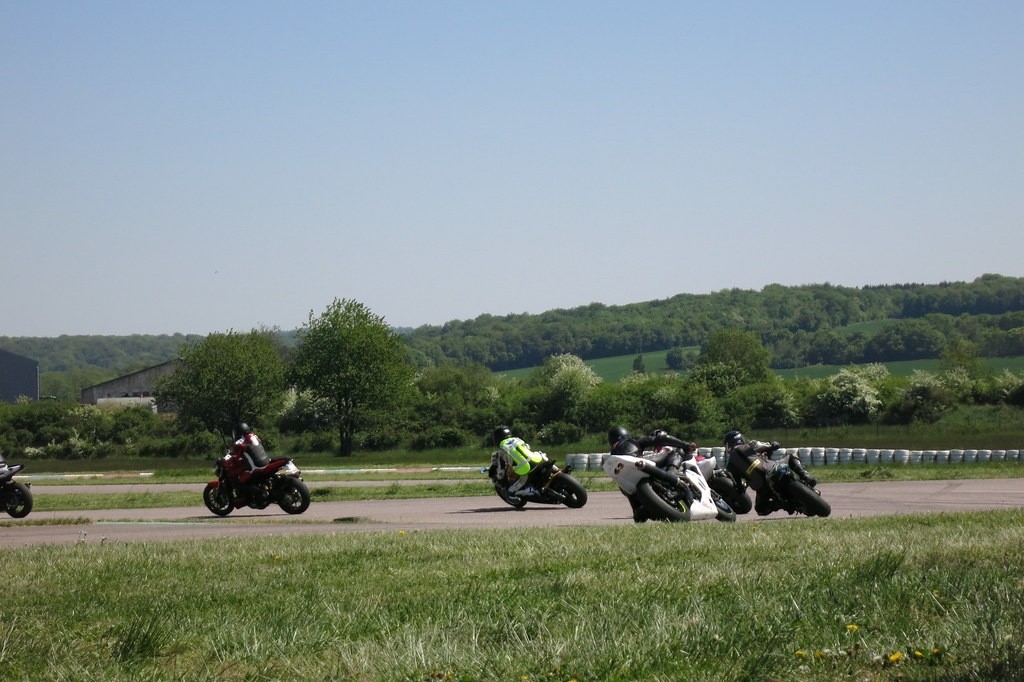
[[234, 423, 251, 440], [608, 426, 630, 445], [724, 430, 746, 449], [494, 425, 512, 444]]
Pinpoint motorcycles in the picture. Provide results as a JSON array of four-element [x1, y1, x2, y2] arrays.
[[602, 443, 752, 522], [203, 428, 310, 516], [0, 461, 33, 518], [481, 451, 587, 507], [741, 441, 831, 517]]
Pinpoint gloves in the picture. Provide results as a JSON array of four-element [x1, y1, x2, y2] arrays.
[[682, 441, 697, 454]]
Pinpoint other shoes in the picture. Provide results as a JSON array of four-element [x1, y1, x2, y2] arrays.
[[766, 497, 786, 514], [799, 468, 817, 487], [665, 465, 690, 484]]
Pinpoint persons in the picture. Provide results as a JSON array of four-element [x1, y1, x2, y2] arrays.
[[608, 426, 696, 524], [231, 422, 270, 506], [723, 431, 816, 516], [493, 426, 561, 499]]
